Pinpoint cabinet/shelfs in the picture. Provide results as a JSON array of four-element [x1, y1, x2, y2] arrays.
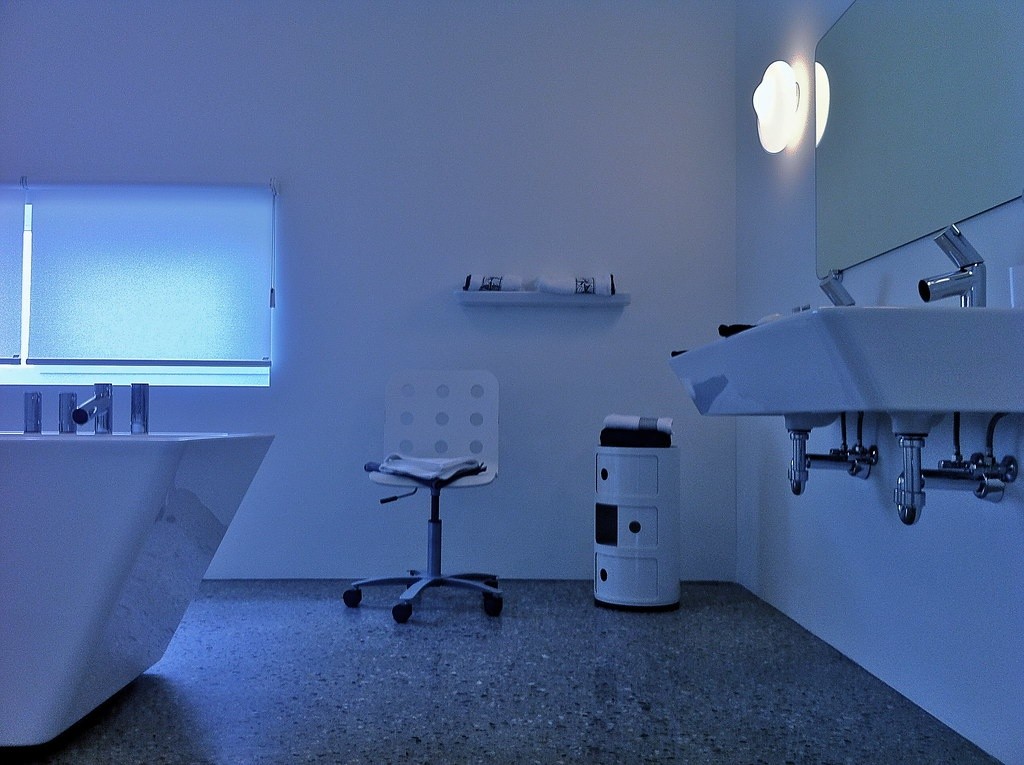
[[592, 443, 681, 613]]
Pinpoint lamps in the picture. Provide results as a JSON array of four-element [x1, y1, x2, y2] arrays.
[[752, 60, 800, 155]]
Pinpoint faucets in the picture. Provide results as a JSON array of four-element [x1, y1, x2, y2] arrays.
[[812, 273, 857, 313], [71, 382, 118, 435], [916, 218, 986, 305]]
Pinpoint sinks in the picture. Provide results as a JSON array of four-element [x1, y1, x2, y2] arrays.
[[670, 295, 1024, 418]]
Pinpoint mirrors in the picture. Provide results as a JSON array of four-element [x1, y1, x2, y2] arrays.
[[814, 0, 1024, 280]]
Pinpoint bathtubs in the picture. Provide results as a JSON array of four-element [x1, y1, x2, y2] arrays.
[[0, 423, 274, 751]]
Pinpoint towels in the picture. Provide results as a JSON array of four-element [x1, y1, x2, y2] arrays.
[[463, 272, 522, 293], [537, 271, 616, 295], [363, 451, 488, 491]]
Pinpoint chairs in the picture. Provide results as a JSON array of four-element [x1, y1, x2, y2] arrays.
[[342, 368, 503, 622]]
